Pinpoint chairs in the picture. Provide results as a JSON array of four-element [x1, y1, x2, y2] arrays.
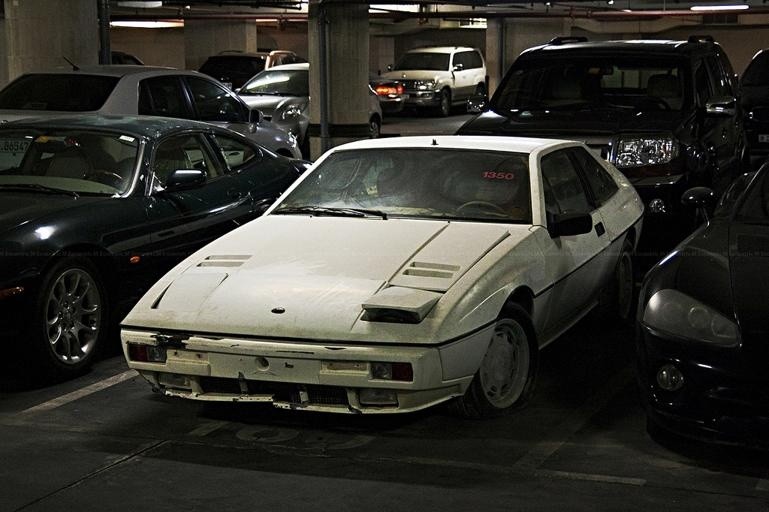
[[542, 73, 600, 106], [49, 152, 195, 185], [646, 75, 680, 100]]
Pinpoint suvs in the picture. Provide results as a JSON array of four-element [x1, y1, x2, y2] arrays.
[[454, 36, 738, 231], [380, 45, 487, 116], [198, 49, 308, 93]]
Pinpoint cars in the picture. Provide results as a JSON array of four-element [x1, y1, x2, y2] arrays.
[[120, 135, 645, 420], [238, 63, 383, 139], [637, 166, 769, 471], [0, 64, 303, 159], [1, 114, 314, 376]]
